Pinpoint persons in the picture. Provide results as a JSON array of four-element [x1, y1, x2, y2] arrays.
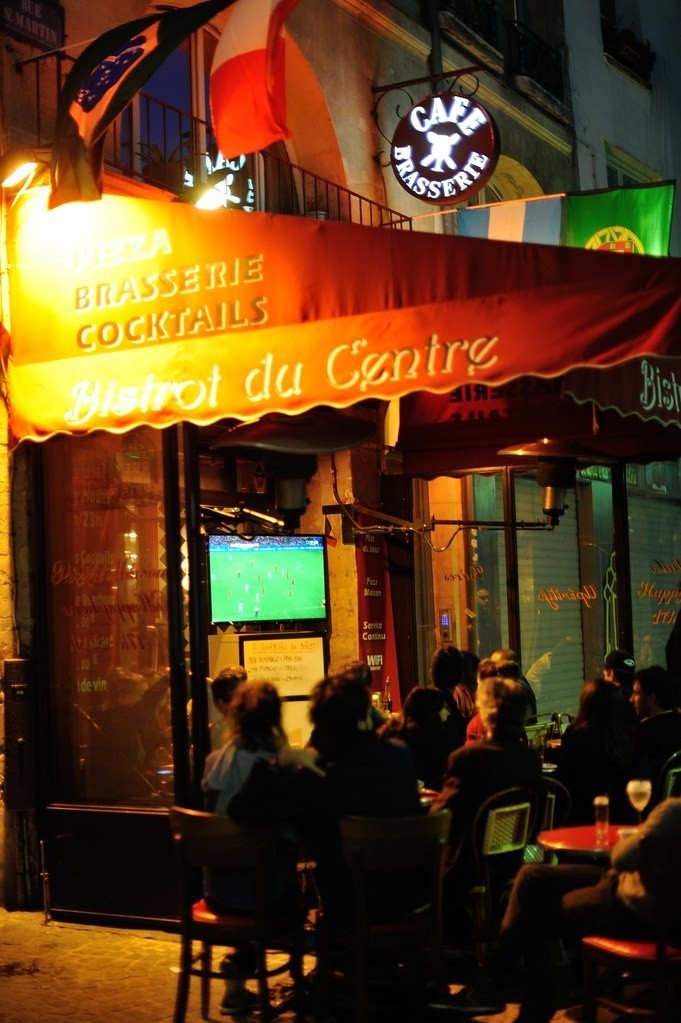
[[430, 798, 681, 1013], [188, 666, 245, 751], [202, 660, 430, 1014], [545, 578, 681, 820], [376, 645, 537, 980]]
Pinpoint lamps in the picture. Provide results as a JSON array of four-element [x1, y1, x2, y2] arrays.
[[196, 407, 379, 541], [432, 435, 618, 531]]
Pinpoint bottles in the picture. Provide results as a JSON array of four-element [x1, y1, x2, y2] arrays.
[[537, 736, 544, 765], [382, 679, 392, 714]]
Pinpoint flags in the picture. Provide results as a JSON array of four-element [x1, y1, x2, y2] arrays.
[[457, 180, 674, 255], [43, 0, 291, 211]]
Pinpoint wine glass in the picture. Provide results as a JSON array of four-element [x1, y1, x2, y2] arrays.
[[625, 781, 652, 830]]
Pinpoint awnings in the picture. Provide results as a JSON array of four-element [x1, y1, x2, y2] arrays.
[[0, 157, 681, 457]]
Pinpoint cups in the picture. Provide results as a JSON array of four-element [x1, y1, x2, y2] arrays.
[[372, 692, 383, 712], [593, 791, 610, 845]]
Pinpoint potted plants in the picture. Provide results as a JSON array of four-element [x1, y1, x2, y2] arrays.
[[136, 136, 207, 189]]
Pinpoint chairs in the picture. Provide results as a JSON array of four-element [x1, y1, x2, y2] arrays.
[[167, 707, 681, 1023]]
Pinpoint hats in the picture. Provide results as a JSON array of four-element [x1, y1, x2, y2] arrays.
[[606, 650, 636, 673]]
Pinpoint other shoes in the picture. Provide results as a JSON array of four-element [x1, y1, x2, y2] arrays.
[[219, 990, 259, 1013], [428, 987, 503, 1012]]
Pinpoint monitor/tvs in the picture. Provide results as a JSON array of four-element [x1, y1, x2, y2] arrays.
[[206, 534, 329, 632]]
